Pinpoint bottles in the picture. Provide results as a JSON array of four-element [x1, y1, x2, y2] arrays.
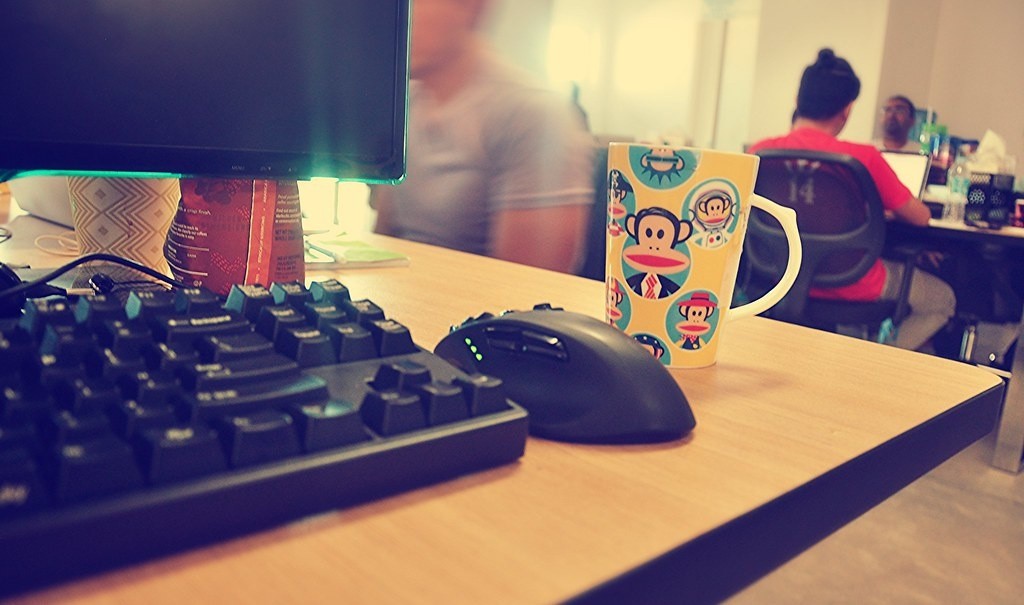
[[942, 156, 971, 225]]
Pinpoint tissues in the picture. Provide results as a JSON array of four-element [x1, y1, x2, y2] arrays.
[[969, 127, 1016, 175]]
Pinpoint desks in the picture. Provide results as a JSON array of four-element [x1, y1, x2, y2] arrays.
[[0, 234, 1010, 605], [925, 215, 1024, 247]]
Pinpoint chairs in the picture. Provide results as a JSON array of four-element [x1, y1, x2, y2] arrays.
[[737, 147, 925, 336]]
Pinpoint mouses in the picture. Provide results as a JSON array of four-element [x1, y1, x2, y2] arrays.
[[434, 304, 695, 445]]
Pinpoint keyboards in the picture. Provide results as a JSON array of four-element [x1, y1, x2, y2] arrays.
[[0, 280, 526, 599]]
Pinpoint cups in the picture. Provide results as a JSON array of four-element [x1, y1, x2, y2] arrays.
[[603, 142, 803, 370], [69, 175, 180, 293]]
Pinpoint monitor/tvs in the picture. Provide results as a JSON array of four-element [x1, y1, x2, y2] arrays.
[[877, 149, 933, 202], [0, 0, 410, 187]]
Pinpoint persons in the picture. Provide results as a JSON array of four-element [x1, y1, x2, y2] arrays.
[[374, 0, 595, 274], [746, 47, 956, 350], [873, 95, 924, 155]]
